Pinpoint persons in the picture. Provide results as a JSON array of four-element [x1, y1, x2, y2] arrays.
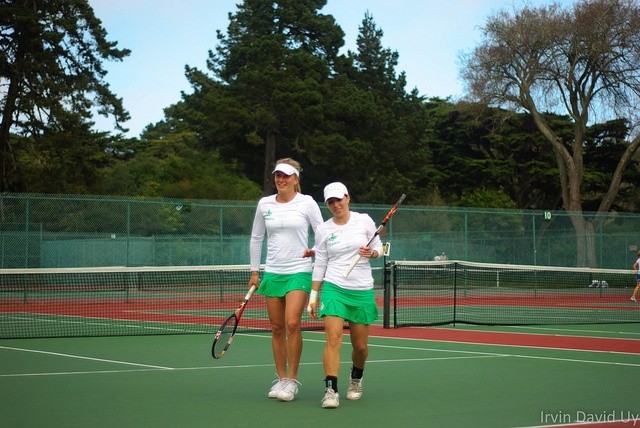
[[440, 251, 448, 261], [631, 251, 639, 303], [434, 254, 440, 261], [306, 181, 383, 409], [247, 157, 324, 400]]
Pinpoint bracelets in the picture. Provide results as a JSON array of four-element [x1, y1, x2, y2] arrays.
[[370, 251, 374, 257]]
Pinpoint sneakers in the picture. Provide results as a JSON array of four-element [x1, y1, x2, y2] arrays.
[[321, 389, 340, 408], [268, 372, 287, 397], [630, 296, 637, 302], [346, 379, 363, 401], [278, 379, 302, 400]]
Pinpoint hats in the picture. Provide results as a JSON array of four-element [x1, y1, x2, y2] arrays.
[[323, 181, 349, 203], [272, 162, 302, 178]]
